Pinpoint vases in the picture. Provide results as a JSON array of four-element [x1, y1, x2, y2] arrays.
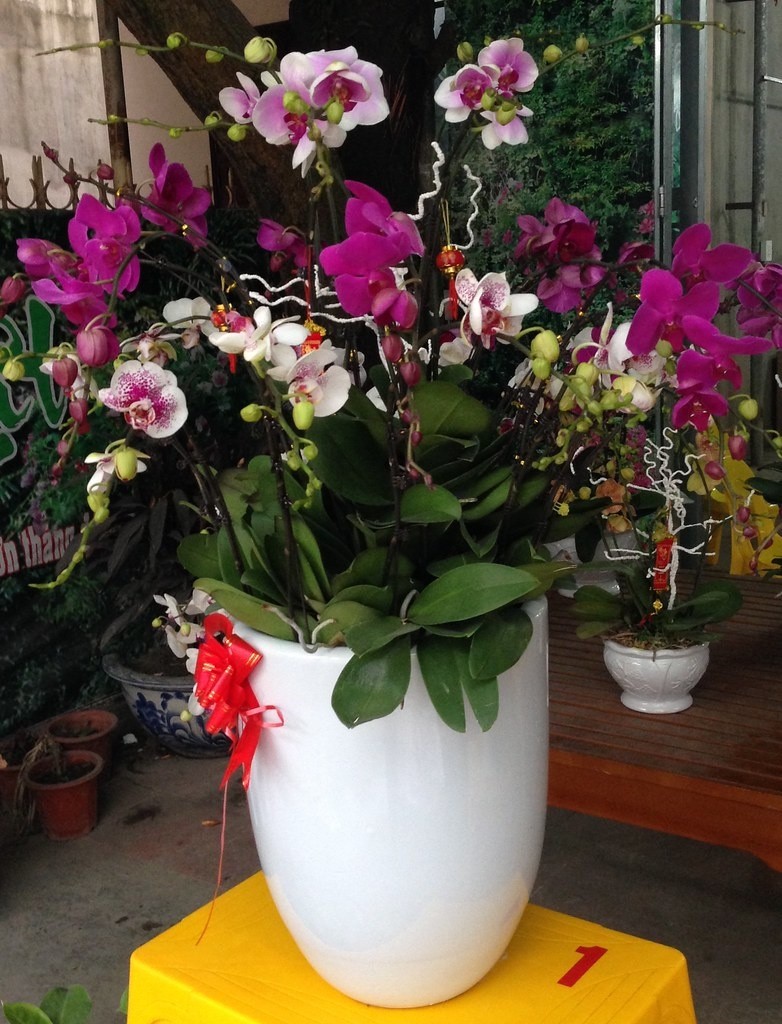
[[602, 638, 710, 714], [50, 711, 118, 770], [0, 738, 21, 803], [99, 604, 232, 760], [230, 594, 552, 1008], [542, 530, 641, 599]]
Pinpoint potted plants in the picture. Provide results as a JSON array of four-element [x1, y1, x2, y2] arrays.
[[13, 735, 104, 841]]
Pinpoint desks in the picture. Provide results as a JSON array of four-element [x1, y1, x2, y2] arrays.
[[543, 572, 782, 873], [126, 868, 696, 1023]]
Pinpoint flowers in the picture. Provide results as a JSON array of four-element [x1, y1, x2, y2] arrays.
[[0, 20, 782, 735]]
